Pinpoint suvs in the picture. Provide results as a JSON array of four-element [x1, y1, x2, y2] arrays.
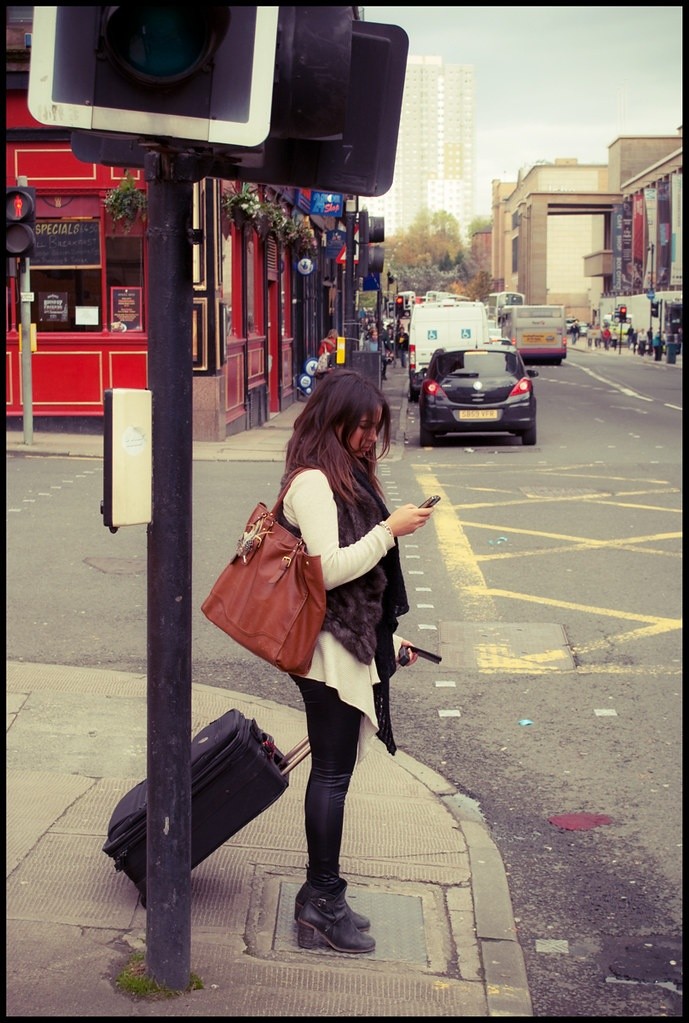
[[419, 345, 539, 448]]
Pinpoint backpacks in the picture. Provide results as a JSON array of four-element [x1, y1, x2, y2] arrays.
[[317, 341, 330, 374]]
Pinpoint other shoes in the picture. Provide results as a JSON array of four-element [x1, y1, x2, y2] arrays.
[[401, 362, 406, 369]]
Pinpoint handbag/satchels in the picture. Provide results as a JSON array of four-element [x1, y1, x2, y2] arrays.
[[635, 345, 639, 349], [644, 344, 649, 351], [199, 467, 327, 674]]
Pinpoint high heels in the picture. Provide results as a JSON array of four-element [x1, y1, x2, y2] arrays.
[[293, 879, 372, 930], [297, 883, 377, 954]]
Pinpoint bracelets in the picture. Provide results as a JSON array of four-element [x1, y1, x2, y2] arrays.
[[380, 521, 394, 541]]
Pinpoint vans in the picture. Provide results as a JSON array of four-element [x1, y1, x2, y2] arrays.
[[407, 301, 492, 400]]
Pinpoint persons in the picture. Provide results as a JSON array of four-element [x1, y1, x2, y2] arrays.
[[319, 308, 406, 382], [569, 316, 667, 358], [275, 367, 435, 954]]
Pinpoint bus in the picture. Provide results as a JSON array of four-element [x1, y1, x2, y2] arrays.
[[488, 292, 524, 327], [502, 303, 568, 364]]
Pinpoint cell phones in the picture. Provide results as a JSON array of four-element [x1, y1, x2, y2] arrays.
[[418, 496, 440, 509]]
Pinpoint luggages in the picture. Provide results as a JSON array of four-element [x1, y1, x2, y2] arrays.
[[100, 646, 409, 911]]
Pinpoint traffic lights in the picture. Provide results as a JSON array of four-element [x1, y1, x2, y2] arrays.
[[356, 209, 387, 280], [617, 307, 627, 322], [394, 296, 405, 319], [4, 187, 35, 261], [651, 301, 659, 318]]
